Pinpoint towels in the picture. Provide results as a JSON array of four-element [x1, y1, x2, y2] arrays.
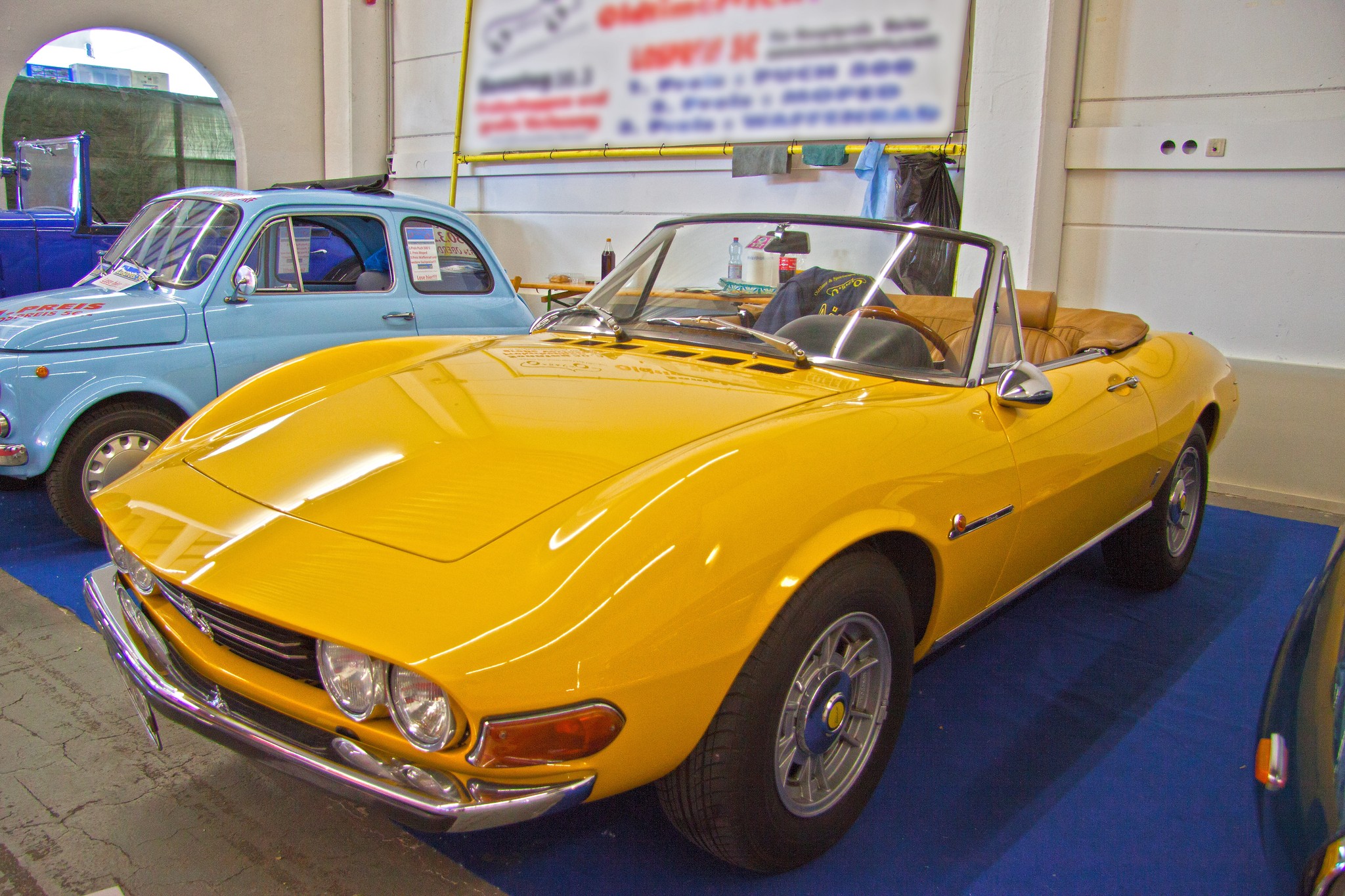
[[856, 140, 889, 220]]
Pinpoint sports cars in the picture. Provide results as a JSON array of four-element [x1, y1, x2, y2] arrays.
[[1252, 522, 1345, 895], [79, 210, 1241, 875]]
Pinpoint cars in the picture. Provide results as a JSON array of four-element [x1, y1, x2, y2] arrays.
[[0, 131, 393, 302], [2, 187, 536, 546]]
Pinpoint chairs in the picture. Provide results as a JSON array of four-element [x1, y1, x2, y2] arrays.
[[758, 266, 886, 348], [353, 271, 392, 292], [945, 287, 1074, 378]]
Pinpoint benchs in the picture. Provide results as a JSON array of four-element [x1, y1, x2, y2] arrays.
[[412, 271, 490, 292], [885, 294, 1149, 354]]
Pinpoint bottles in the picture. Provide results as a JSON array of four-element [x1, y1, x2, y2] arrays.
[[601, 238, 616, 280], [779, 253, 797, 283], [728, 237, 742, 281]]
